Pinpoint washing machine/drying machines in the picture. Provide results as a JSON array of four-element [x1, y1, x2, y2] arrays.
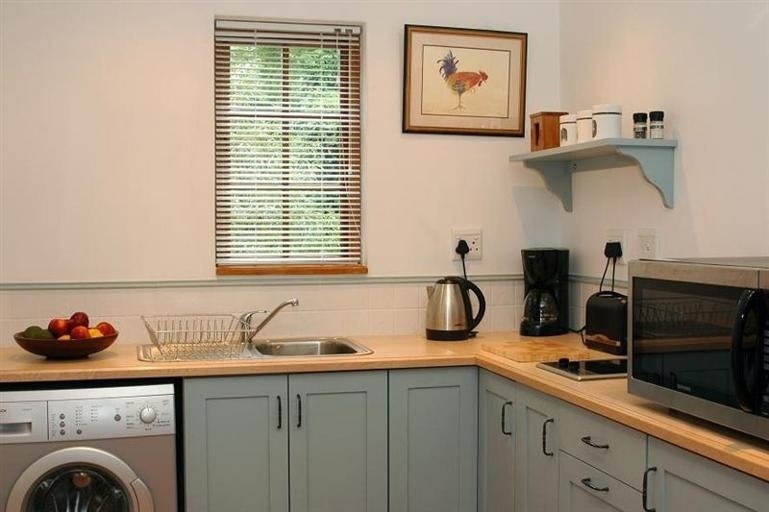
[[0, 381, 178, 511]]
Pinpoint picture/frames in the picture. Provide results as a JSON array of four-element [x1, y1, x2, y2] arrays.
[[403, 24, 527, 139]]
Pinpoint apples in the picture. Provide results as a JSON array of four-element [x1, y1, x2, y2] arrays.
[[48, 311, 116, 339]]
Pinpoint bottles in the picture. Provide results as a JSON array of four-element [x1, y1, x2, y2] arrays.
[[558, 104, 666, 146]]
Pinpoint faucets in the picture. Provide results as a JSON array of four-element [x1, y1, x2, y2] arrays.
[[238, 297, 299, 342]]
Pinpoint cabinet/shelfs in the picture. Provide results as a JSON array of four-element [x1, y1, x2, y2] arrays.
[[643, 435, 768, 512], [475, 371, 560, 512], [180, 369, 389, 512], [557, 399, 644, 512], [388, 365, 478, 511]]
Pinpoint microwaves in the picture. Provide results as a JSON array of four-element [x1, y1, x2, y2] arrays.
[[623, 254, 768, 443]]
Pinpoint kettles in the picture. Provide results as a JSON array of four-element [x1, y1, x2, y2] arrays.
[[421, 275, 486, 341]]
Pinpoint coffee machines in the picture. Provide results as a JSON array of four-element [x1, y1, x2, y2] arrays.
[[516, 247, 571, 336]]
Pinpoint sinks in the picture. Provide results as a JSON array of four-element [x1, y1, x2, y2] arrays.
[[252, 336, 374, 360]]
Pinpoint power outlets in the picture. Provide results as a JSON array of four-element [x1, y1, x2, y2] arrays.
[[449, 226, 484, 263]]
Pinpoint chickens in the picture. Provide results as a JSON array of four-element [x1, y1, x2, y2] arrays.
[[437, 48, 488, 113]]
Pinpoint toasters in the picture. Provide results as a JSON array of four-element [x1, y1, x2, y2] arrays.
[[586, 289, 626, 356]]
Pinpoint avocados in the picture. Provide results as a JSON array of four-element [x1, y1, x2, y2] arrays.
[[22, 326, 44, 339], [35, 328, 55, 339]]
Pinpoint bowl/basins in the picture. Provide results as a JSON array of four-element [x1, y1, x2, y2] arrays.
[[13, 332, 120, 360]]
